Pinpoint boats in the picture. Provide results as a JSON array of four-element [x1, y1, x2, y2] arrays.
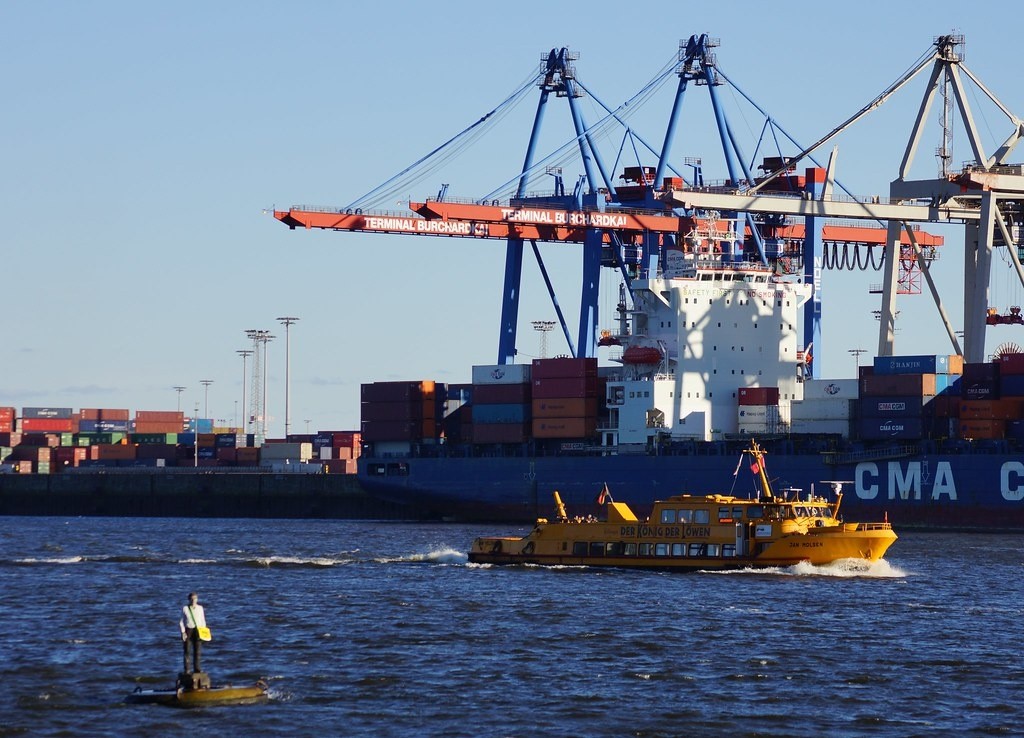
[[124, 670, 270, 709], [467, 436, 900, 572]]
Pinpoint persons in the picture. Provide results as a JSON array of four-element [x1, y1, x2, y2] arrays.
[[180, 593, 206, 673]]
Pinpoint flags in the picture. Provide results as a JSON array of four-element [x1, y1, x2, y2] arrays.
[[750, 457, 765, 474], [594, 487, 608, 506], [733, 454, 743, 475]]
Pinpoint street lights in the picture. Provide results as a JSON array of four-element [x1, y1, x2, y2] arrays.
[[193, 401, 201, 467]]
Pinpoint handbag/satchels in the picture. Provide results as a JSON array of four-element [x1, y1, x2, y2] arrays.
[[196, 626, 212, 642]]
